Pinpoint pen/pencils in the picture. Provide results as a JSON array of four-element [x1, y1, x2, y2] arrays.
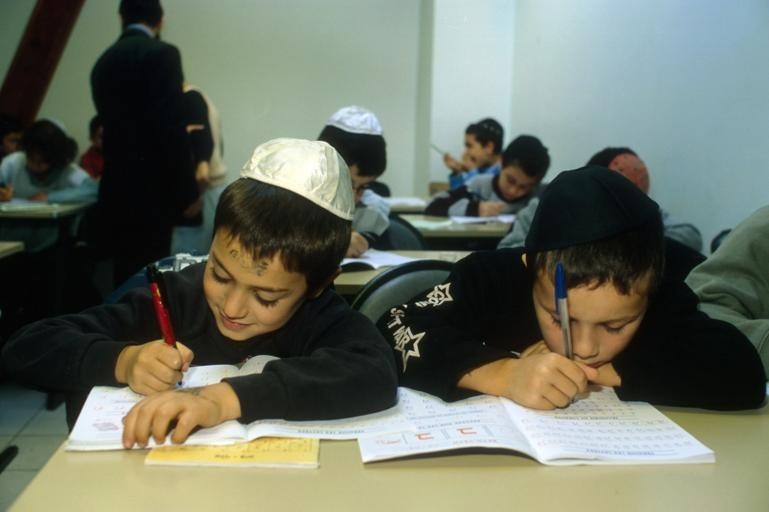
[[430, 143, 446, 156], [475, 193, 488, 201], [146, 262, 183, 389], [555, 261, 572, 361]]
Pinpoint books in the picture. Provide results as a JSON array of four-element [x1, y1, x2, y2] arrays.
[[341, 249, 411, 270], [66, 355, 398, 452], [357, 387, 715, 467], [144, 437, 321, 469]]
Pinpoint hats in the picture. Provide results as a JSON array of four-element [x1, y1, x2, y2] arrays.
[[524, 167, 658, 252], [329, 105, 381, 137], [241, 137, 355, 221], [480, 117, 502, 140]]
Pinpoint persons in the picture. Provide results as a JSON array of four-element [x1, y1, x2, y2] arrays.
[[376, 166, 765, 411], [1, 1, 226, 298], [498, 147, 701, 251], [1, 136, 398, 451], [421, 135, 550, 250], [317, 106, 391, 259], [429, 118, 504, 197], [684, 205, 768, 384]]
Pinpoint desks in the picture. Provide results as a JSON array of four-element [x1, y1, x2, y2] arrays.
[[0, 195, 769, 512]]
